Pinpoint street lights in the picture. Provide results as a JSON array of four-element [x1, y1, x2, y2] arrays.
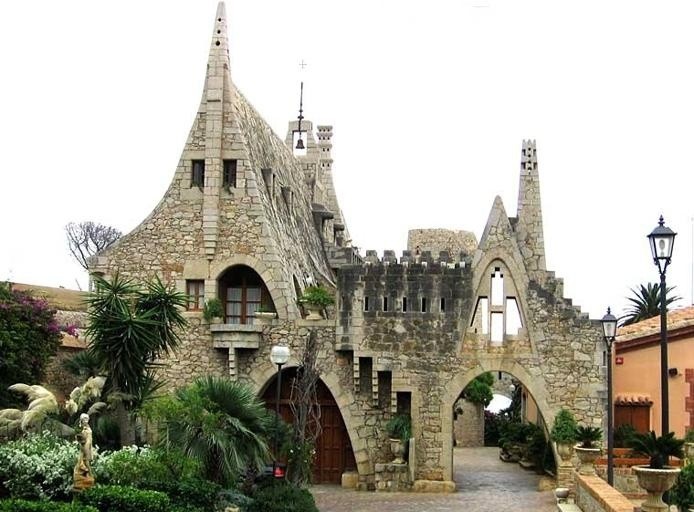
[[597, 306, 622, 487], [269, 340, 292, 483], [644, 214, 678, 465]]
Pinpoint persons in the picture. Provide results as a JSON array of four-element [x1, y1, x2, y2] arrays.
[[79, 412, 93, 461]]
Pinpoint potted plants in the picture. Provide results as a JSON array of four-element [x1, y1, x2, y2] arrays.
[[629, 429, 688, 512], [384, 410, 414, 463], [202, 298, 225, 324], [548, 407, 604, 477], [292, 282, 336, 321], [252, 303, 276, 326]]
